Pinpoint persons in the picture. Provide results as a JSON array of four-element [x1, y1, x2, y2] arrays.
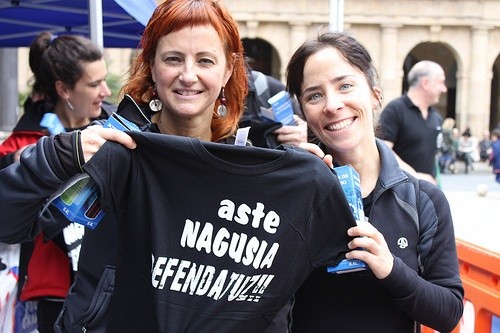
[[0, 0, 295, 333], [376, 61, 447, 186], [434, 118, 500, 182], [285, 32, 464, 333], [0, 32, 308, 333]]
